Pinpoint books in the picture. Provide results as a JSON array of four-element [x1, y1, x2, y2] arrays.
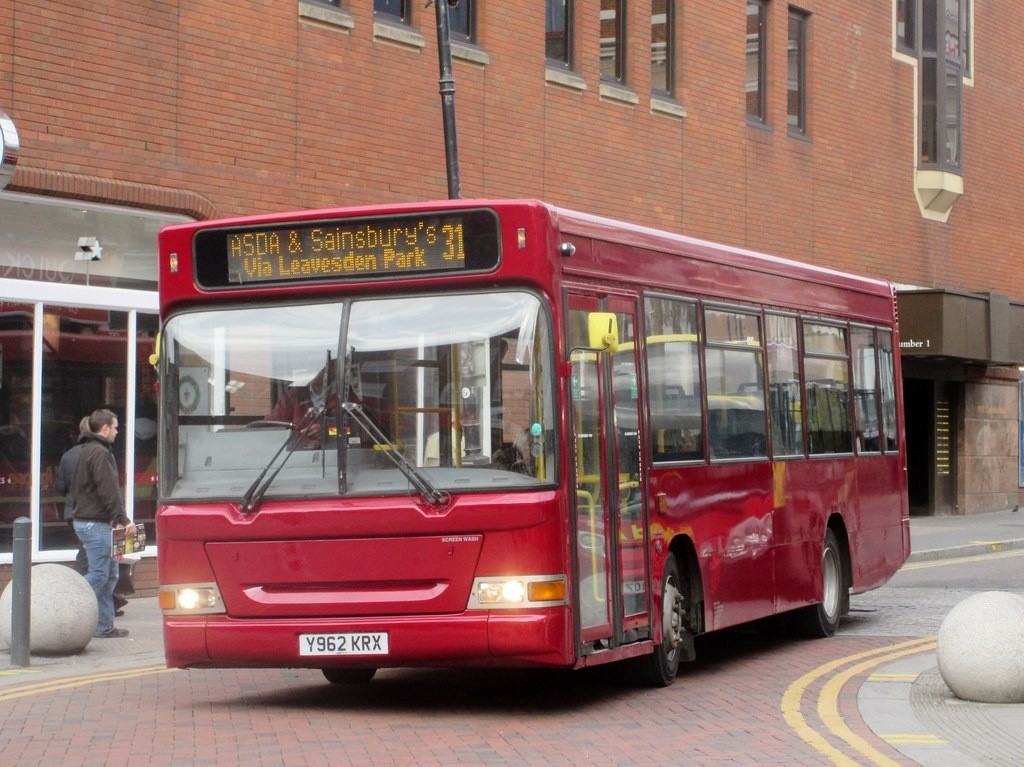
[[111, 523, 146, 556]]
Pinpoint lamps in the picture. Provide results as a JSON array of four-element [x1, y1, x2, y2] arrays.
[[77, 237, 102, 288]]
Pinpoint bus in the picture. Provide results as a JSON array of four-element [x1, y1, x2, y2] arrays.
[[158, 196, 914, 691], [349, 359, 481, 448], [567, 333, 806, 602], [0, 328, 158, 541]]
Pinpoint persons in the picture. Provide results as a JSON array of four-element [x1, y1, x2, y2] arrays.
[[262, 349, 372, 449], [55, 417, 128, 617], [72, 410, 137, 638]]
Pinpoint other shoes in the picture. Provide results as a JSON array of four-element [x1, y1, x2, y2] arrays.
[[115, 609, 125, 618], [93, 629, 130, 637]]
